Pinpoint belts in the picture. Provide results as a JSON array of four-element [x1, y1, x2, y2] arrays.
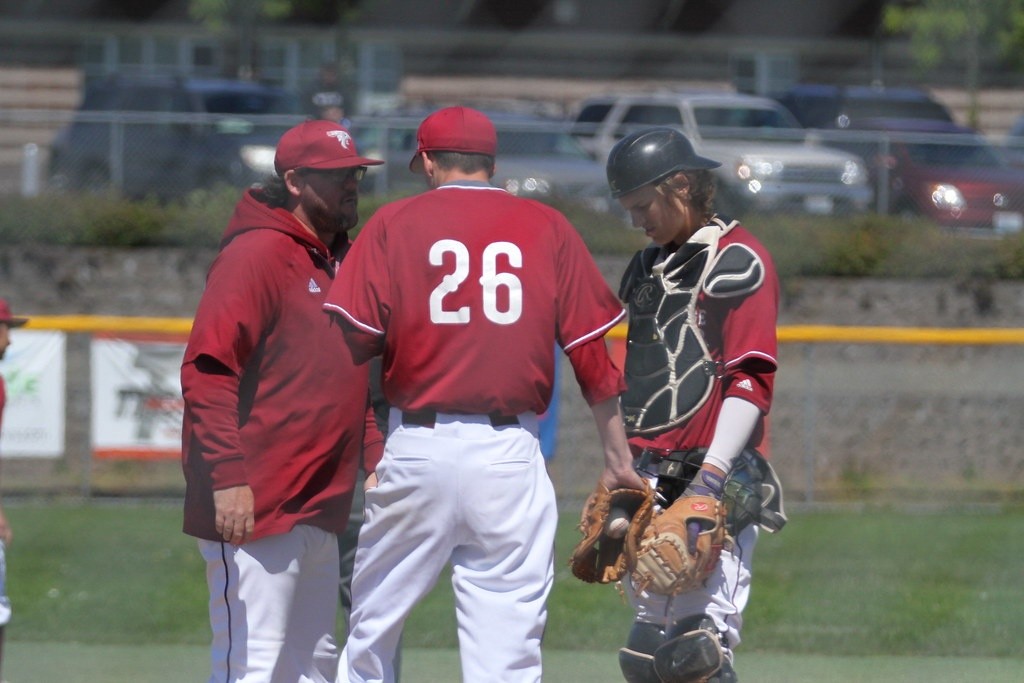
[[402, 409, 521, 427]]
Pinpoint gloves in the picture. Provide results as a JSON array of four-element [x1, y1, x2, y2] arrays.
[[674, 469, 724, 555]]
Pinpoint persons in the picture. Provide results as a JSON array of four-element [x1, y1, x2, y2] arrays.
[[607, 126, 788, 683], [0, 296, 29, 683], [180, 120, 389, 682], [323, 105, 646, 683]]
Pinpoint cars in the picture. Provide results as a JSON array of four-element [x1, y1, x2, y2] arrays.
[[352, 109, 625, 228]]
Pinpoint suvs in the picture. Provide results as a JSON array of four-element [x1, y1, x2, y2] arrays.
[[757, 83, 1024, 232], [50, 68, 326, 197], [556, 88, 875, 226]]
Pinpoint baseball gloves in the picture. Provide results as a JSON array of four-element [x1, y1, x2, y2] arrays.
[[568, 484, 653, 584], [630, 495, 730, 596]]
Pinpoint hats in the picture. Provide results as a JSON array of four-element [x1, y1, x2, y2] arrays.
[[409, 105, 497, 172], [275, 118, 385, 176]]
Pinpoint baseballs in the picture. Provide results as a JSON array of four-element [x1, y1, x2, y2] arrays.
[[603, 506, 630, 540]]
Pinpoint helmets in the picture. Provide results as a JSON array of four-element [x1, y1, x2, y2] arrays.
[[607, 127, 723, 199]]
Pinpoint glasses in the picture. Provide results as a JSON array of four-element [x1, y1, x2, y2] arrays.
[[297, 166, 367, 183]]
